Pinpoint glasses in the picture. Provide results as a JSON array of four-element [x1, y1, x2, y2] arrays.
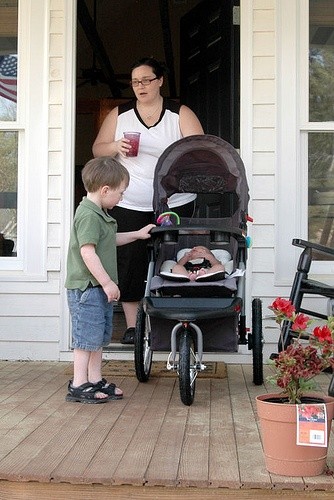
[[128, 77, 158, 87]]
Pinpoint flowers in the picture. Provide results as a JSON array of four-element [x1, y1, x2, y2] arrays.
[[266, 298, 334, 404]]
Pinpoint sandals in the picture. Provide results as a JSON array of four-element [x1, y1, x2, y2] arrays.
[[94, 378, 123, 399], [65, 380, 108, 404]]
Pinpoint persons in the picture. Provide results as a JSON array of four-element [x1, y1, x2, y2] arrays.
[[65, 157, 156, 403], [171, 245, 225, 281], [92, 57, 204, 344]]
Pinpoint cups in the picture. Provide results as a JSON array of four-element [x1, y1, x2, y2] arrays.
[[123, 131, 141, 158]]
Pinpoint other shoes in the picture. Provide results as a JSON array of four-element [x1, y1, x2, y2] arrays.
[[120, 327, 136, 343], [148, 331, 151, 346]]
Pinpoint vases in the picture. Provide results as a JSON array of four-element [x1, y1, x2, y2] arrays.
[[255, 392, 334, 476]]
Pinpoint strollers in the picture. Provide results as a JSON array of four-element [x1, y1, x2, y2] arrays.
[[133, 136, 264, 407]]
